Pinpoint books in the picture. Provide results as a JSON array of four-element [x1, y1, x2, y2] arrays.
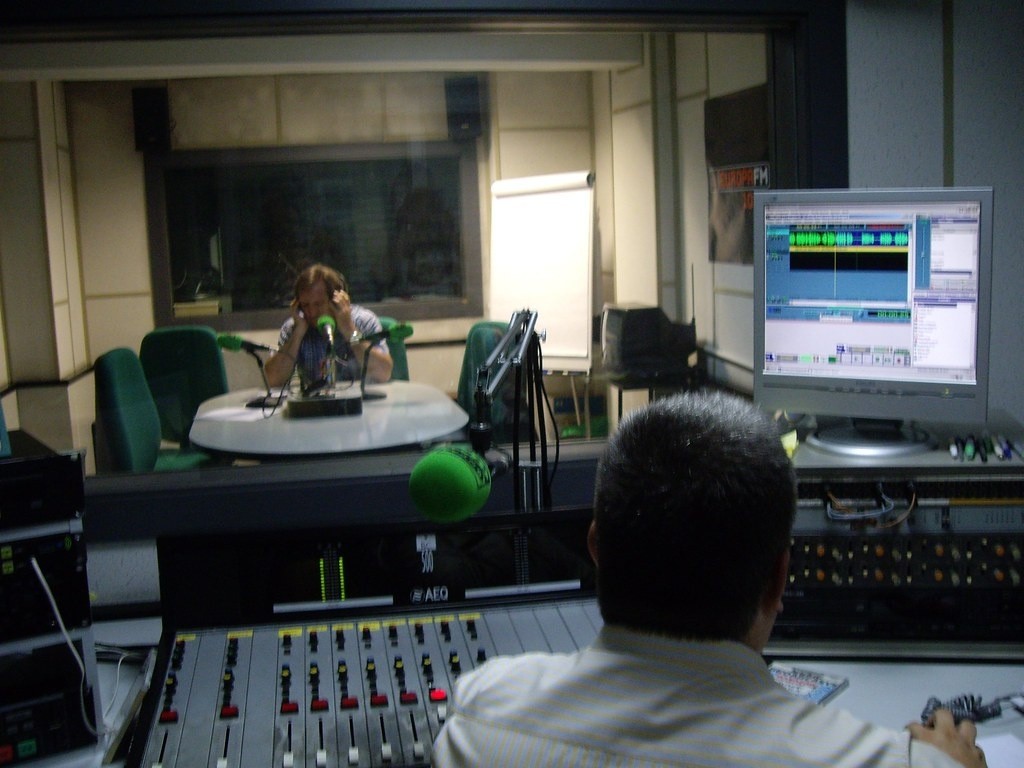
[[767, 661, 849, 706]]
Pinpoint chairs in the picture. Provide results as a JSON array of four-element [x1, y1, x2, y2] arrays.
[[452, 321, 526, 462], [138, 325, 237, 468], [379, 317, 410, 381], [455, 327, 502, 442], [92, 346, 204, 480]]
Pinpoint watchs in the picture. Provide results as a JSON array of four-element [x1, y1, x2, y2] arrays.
[[349, 330, 362, 342]]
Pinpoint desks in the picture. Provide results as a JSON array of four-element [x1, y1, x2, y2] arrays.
[[187, 381, 470, 462]]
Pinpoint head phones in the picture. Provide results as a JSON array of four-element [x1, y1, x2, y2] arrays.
[[295, 275, 347, 320]]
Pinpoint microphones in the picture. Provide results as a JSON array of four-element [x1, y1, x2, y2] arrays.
[[218, 335, 270, 352], [317, 314, 336, 346], [359, 323, 414, 343], [408, 441, 508, 525]]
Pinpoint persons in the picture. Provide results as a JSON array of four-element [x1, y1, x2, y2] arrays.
[[264, 264, 393, 387], [431, 393, 988, 768]]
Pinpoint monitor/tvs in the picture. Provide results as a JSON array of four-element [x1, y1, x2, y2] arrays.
[[746, 185, 997, 461], [598, 304, 694, 385]]
